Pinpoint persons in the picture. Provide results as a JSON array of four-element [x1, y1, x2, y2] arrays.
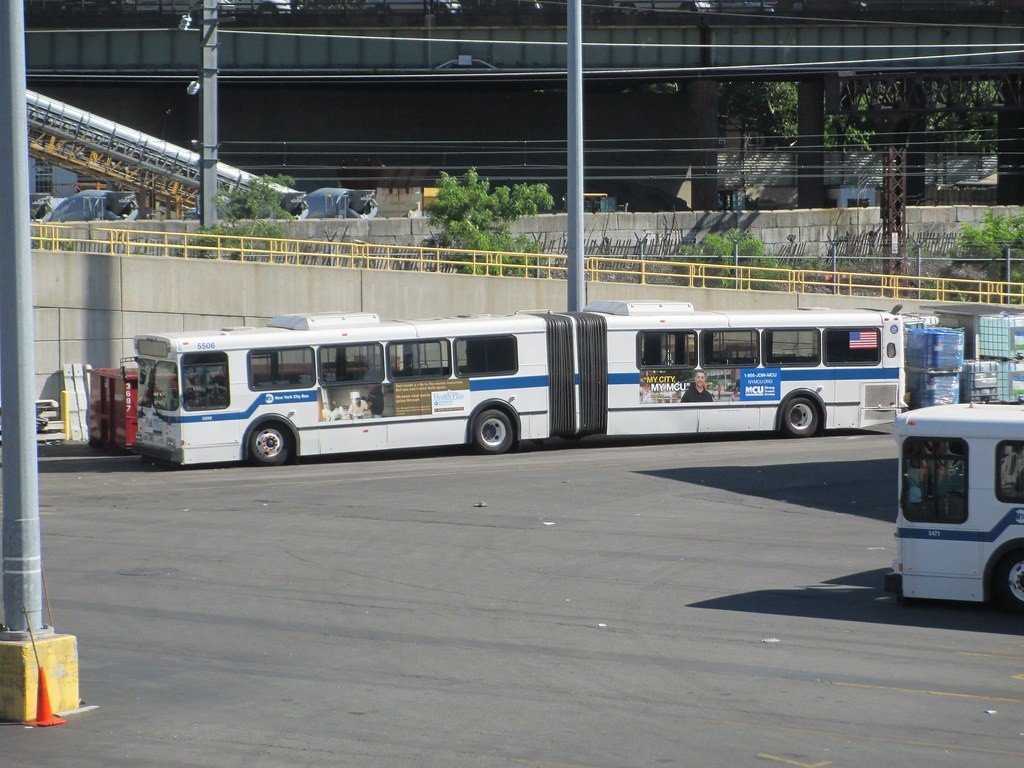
[[680, 369, 715, 403], [593, 200, 600, 213], [321, 384, 385, 421], [397, 353, 413, 376]]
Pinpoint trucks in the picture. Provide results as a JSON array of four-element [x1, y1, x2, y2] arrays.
[[287, 187, 378, 219], [35, 189, 138, 226]]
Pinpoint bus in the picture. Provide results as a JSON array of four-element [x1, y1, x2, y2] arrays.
[[133, 310, 907, 467], [893, 403, 1024, 615]]
